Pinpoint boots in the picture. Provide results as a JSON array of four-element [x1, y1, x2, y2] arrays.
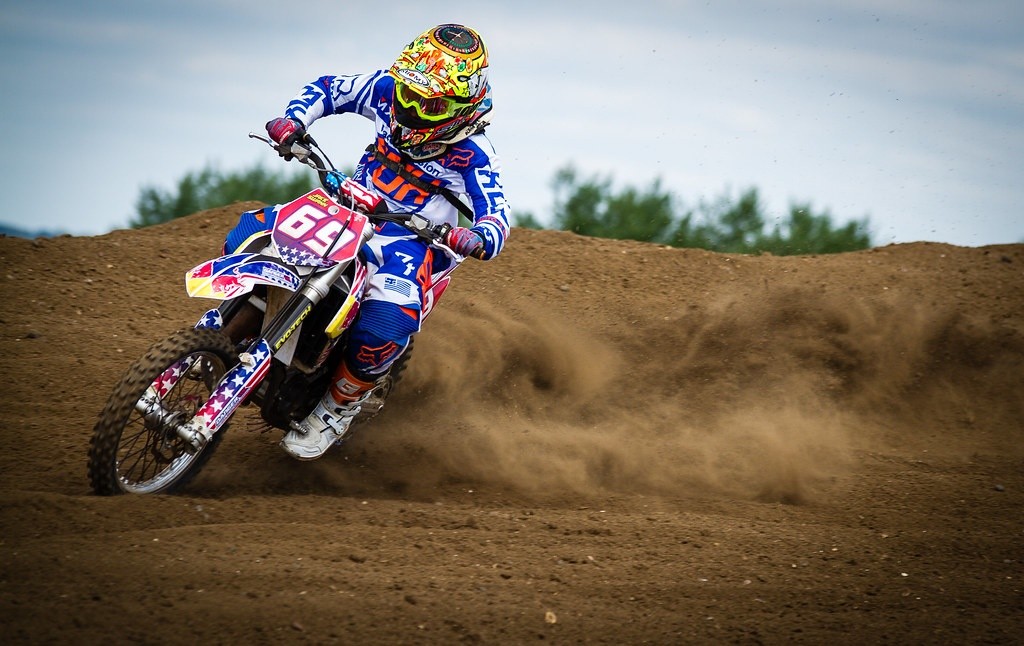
[[278, 360, 390, 461]]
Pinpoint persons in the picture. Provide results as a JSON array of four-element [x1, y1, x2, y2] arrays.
[[226, 23, 510, 462]]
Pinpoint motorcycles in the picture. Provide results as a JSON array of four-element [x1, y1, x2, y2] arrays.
[[81, 121, 497, 497]]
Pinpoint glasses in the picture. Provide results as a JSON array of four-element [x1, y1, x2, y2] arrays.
[[393, 78, 474, 122]]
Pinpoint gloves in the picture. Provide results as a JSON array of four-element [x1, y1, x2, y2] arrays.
[[267, 117, 302, 145], [446, 227, 484, 257]]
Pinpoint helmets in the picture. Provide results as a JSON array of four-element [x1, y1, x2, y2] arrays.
[[388, 24, 490, 149]]
